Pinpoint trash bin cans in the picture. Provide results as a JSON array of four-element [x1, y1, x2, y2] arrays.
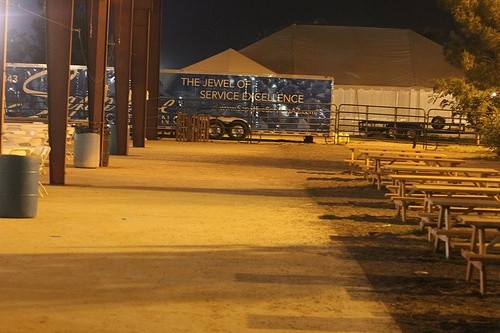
[[0, 147, 37, 218], [73, 126, 101, 168]]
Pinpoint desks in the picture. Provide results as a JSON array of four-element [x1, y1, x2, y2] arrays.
[[345, 142, 500, 298]]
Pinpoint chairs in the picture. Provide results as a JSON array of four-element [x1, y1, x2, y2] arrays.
[[2, 121, 76, 196]]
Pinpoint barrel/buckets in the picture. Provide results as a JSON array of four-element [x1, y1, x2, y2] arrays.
[[0, 154, 41, 219]]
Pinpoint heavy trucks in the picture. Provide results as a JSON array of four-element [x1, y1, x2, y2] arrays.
[[0, 61, 333, 141]]
